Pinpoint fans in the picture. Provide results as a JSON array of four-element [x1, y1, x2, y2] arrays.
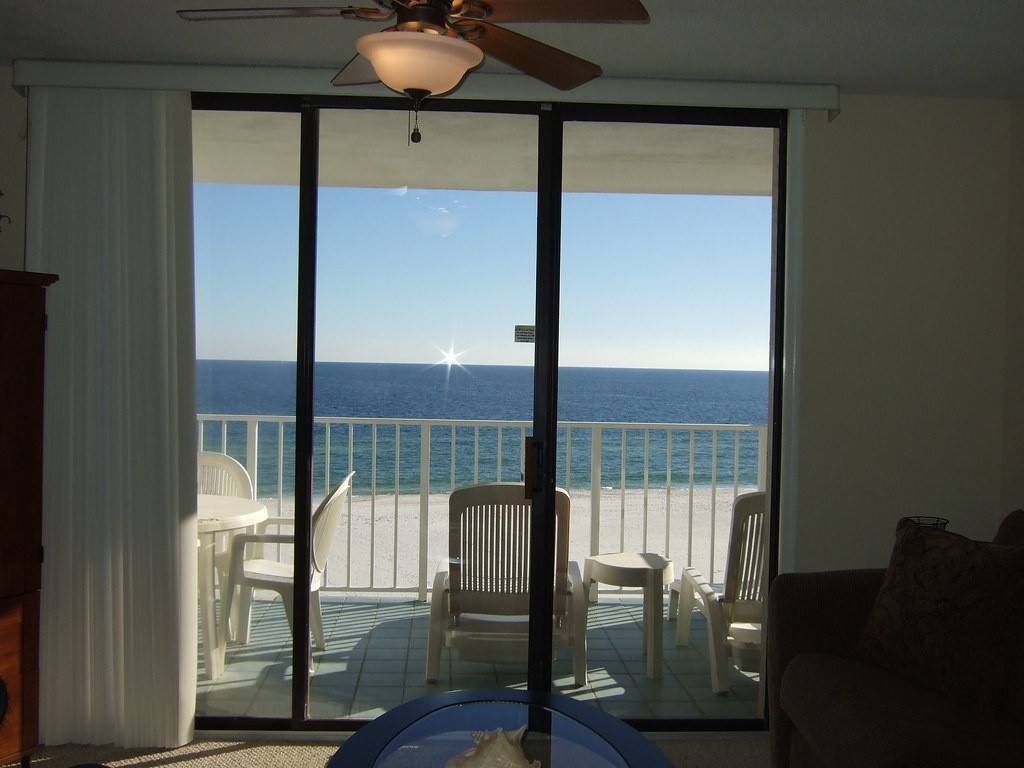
[[175, 0, 653, 91]]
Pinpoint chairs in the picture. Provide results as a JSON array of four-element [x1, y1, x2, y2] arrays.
[[218, 470, 358, 668], [197, 450, 256, 643], [670, 490, 765, 693], [425, 481, 585, 687]]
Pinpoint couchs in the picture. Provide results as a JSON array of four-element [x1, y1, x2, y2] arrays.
[[766, 508, 1024, 768]]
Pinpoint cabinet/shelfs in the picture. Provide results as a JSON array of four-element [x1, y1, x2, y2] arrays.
[[0, 266, 59, 768]]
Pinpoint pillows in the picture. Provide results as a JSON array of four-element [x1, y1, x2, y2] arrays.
[[851, 517, 1024, 716]]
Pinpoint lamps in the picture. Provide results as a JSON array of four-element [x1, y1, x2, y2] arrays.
[[356, 30, 485, 148]]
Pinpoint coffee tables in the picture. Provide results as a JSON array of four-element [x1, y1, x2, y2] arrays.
[[326, 688, 676, 768]]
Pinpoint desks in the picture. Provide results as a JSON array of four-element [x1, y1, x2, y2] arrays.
[[197, 495, 269, 680], [585, 550, 668, 679]]
